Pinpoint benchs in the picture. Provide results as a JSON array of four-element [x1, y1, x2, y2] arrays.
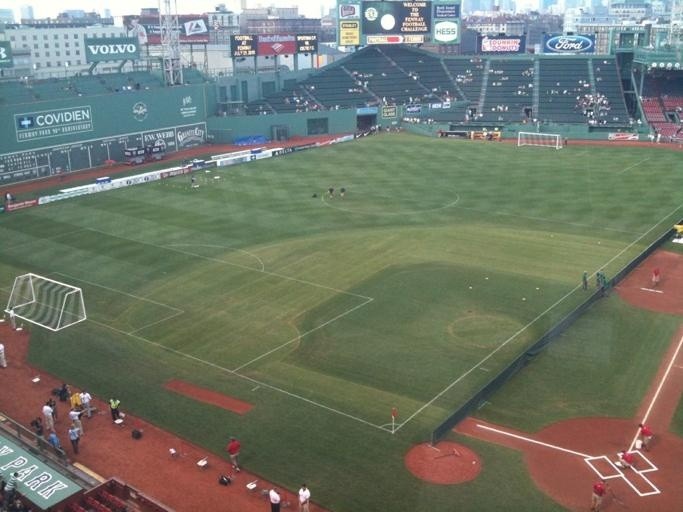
[[0, 66, 208, 109], [245, 43, 682, 142]]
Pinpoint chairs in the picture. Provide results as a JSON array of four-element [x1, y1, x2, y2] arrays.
[[0, 482, 129, 512], [114, 413, 257, 493]]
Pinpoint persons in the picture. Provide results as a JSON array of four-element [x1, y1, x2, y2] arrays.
[[298, 482, 312, 512], [590, 478, 608, 512], [340, 187, 345, 197], [223, 436, 242, 473], [329, 184, 335, 197], [582, 269, 608, 297], [0, 382, 121, 512], [619, 447, 636, 469], [0, 343, 8, 369], [652, 266, 661, 287], [638, 423, 655, 452], [268, 486, 281, 512], [8, 305, 19, 331]]
